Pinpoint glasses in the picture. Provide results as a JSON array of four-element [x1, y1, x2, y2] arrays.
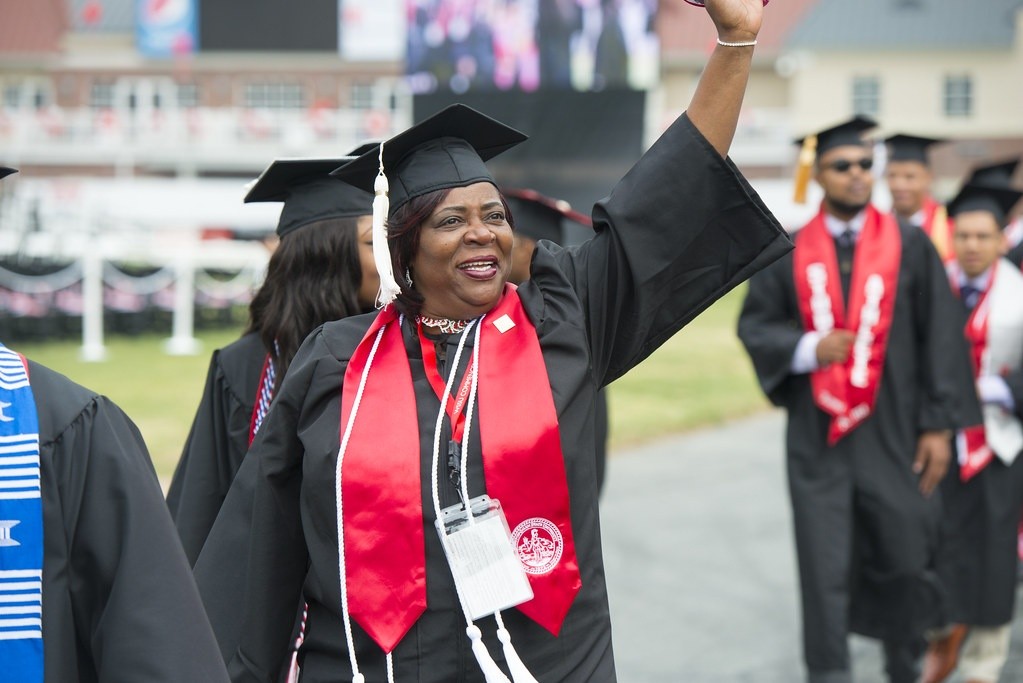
[[817, 157, 873, 173]]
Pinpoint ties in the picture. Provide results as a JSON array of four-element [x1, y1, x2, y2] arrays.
[[961, 287, 974, 301], [842, 229, 856, 244]]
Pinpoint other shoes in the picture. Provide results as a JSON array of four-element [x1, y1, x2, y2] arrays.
[[918, 622, 970, 683]]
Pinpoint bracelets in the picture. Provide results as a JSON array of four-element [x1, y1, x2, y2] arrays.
[[717, 39, 757, 46]]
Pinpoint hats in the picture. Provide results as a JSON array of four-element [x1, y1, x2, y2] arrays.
[[499, 182, 594, 245], [243, 152, 376, 241], [345, 143, 379, 156], [791, 116, 879, 203], [326, 103, 525, 310], [0, 166, 18, 179], [879, 135, 942, 166], [944, 159, 1023, 228]]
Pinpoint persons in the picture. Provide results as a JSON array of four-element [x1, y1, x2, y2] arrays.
[[503, 188, 593, 286], [851, 134, 1023, 683], [736, 114, 984, 683], [166, 160, 384, 568], [191, 0, 797, 683], [0, 342, 230, 683]]
